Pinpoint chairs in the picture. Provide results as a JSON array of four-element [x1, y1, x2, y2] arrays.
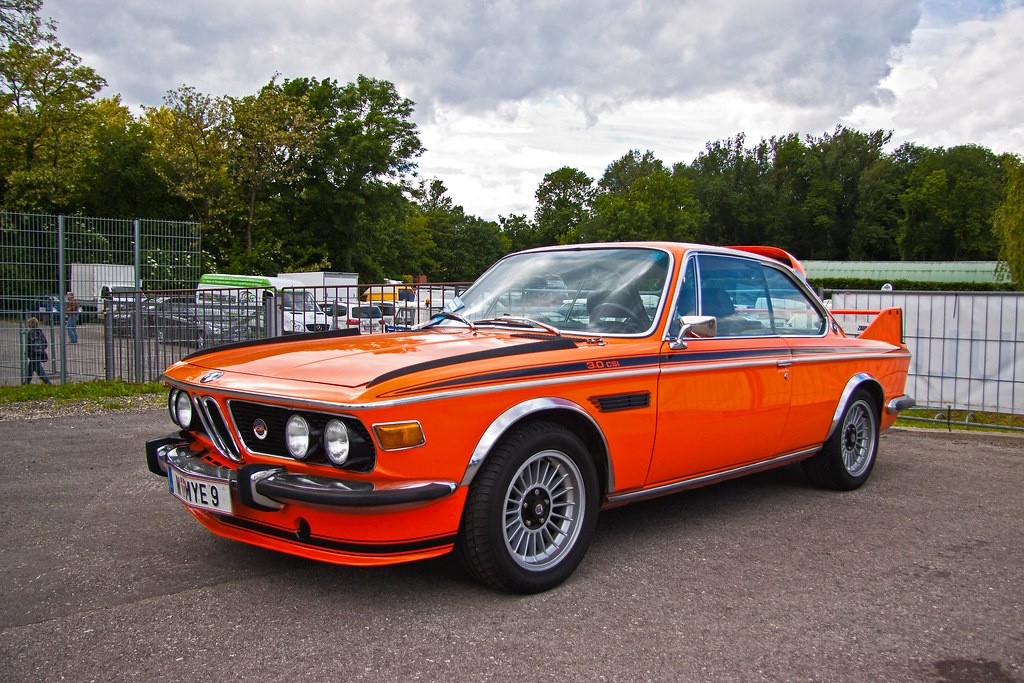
[[586, 290, 633, 318], [678, 288, 734, 319]]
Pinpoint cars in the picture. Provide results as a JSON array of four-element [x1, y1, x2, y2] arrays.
[[35, 295, 78, 325], [733, 297, 833, 330], [539, 295, 681, 325], [143, 242, 917, 595], [153, 302, 250, 348]]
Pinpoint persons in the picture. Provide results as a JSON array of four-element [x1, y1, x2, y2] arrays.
[[22, 318, 54, 384], [64, 292, 80, 343]]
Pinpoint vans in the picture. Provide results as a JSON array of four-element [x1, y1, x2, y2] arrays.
[[196, 274, 335, 335], [360, 286, 414, 301], [114, 295, 195, 338], [370, 302, 403, 326], [414, 286, 508, 329]]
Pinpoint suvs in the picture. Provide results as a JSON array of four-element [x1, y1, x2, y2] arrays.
[[318, 300, 386, 336]]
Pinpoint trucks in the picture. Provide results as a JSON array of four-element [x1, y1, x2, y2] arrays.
[[71, 263, 149, 322], [277, 272, 358, 303]]
